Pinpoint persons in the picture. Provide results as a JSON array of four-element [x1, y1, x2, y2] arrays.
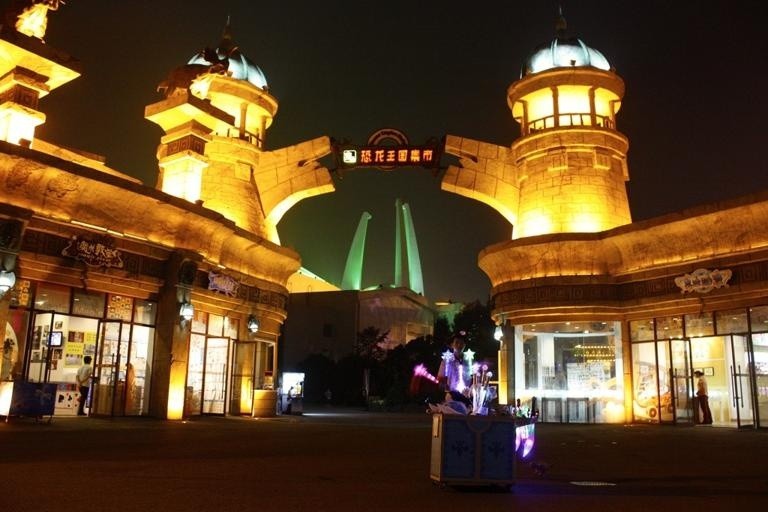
[[74, 355, 92, 416], [694, 370, 713, 424], [286, 386, 294, 401], [433, 329, 475, 404]]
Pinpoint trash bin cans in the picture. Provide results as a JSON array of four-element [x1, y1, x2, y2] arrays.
[[252, 388, 277, 417], [428, 413, 516, 492]]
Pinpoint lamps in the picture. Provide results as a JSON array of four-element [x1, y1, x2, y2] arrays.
[[248, 316, 261, 334], [0, 269, 18, 297], [180, 301, 196, 321]]
